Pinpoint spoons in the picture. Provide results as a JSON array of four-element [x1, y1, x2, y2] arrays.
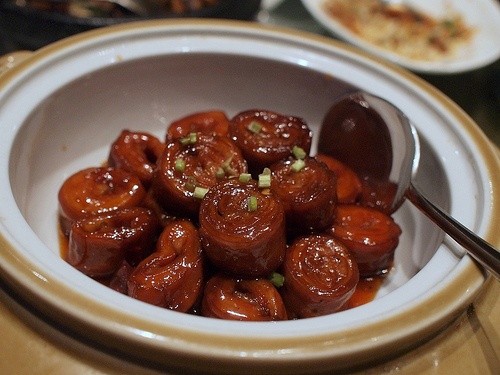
[[317, 91, 500, 284]]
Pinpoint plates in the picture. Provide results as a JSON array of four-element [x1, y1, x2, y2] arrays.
[[301, 1, 499, 74]]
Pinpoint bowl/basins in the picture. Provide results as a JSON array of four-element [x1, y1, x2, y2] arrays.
[[0, 18, 500, 375]]
